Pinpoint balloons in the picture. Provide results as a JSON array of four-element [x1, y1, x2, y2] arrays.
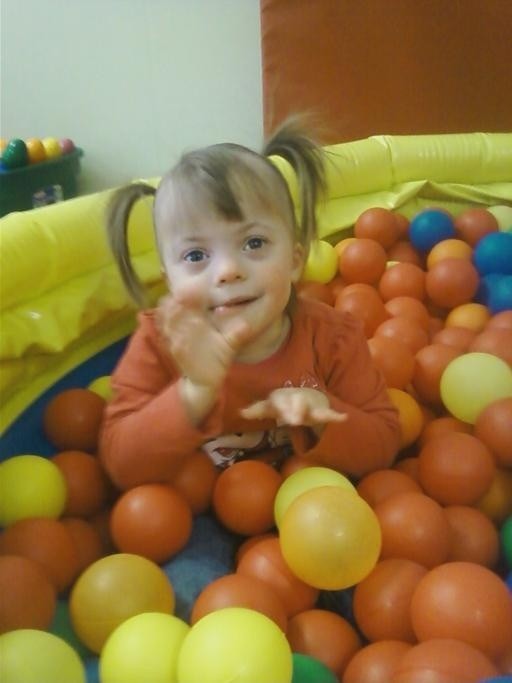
[[0, 131, 80, 170]]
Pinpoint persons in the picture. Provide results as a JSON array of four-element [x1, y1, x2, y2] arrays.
[[100, 110, 403, 493], [100, 110, 403, 628]]
